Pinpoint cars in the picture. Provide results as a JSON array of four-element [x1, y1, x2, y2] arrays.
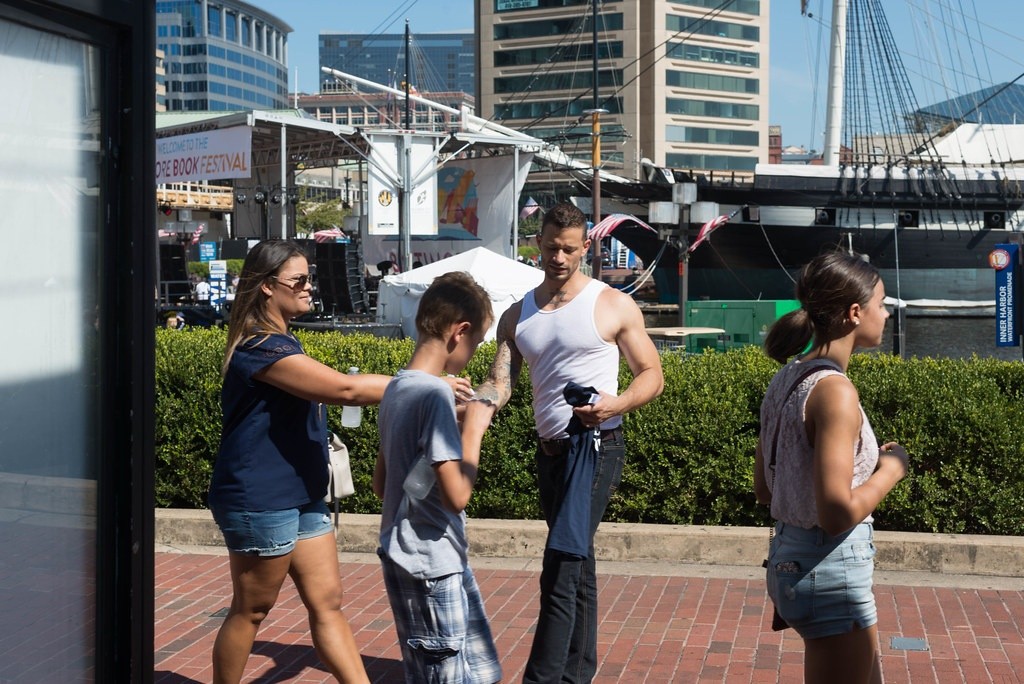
[[589, 245, 611, 268], [156, 305, 228, 330], [157, 279, 198, 301]]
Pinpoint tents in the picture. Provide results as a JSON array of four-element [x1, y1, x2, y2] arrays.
[[373, 245, 547, 348]]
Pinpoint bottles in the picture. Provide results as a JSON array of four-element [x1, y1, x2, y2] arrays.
[[341, 367, 361, 427], [403, 454, 437, 499]]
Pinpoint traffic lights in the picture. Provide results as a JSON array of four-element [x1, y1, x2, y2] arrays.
[[162, 206, 172, 216]]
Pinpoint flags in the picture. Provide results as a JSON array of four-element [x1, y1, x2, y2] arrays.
[[691, 215, 730, 251], [519, 199, 537, 221], [586, 211, 651, 242], [314, 227, 345, 243], [190, 223, 206, 244]]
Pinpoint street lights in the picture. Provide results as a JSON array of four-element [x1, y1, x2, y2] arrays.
[[648, 176, 719, 327], [638, 156, 673, 174], [581, 106, 612, 283]]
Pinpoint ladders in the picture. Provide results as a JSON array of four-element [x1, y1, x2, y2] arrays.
[[345, 243, 370, 314]]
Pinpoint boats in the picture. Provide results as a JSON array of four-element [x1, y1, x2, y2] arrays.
[[290, 318, 402, 338], [903, 117, 1024, 163], [553, 0, 1024, 302], [880, 293, 997, 317]]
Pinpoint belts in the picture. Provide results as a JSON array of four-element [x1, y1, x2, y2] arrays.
[[533, 426, 623, 455]]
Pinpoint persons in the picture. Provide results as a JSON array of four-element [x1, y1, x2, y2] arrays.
[[209, 240, 473, 684], [189, 272, 211, 303], [754, 254, 908, 684], [455, 204, 665, 684], [371, 270, 504, 684]]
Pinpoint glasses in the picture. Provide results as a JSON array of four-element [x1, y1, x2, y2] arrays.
[[271, 273, 312, 294]]
